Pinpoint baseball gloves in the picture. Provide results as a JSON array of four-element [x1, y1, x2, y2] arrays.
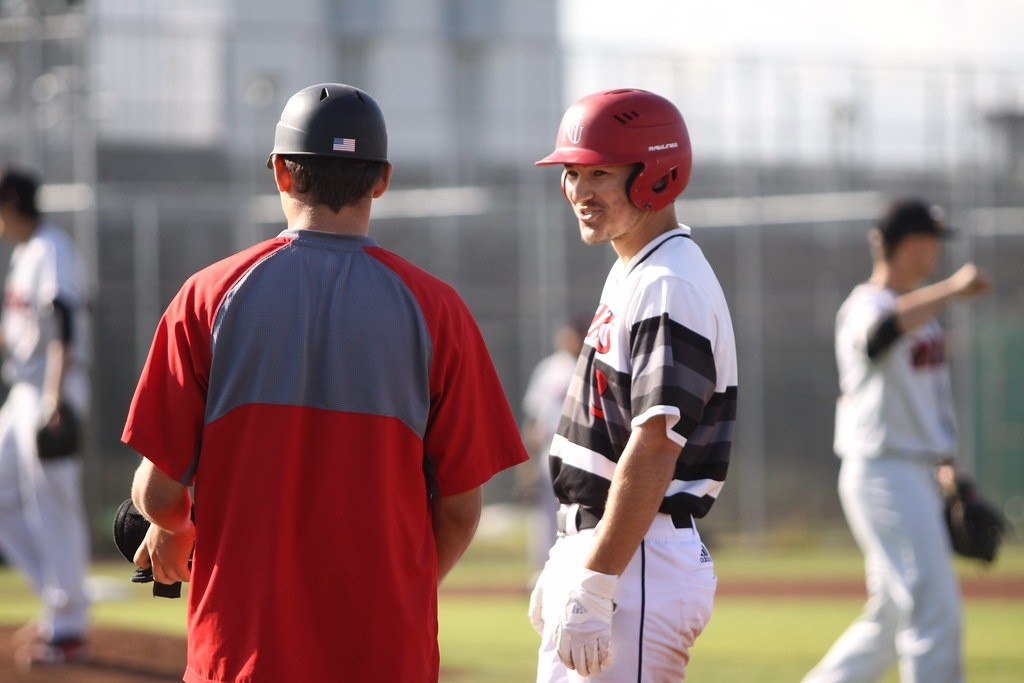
[[34, 400, 85, 465], [942, 480, 1008, 566]]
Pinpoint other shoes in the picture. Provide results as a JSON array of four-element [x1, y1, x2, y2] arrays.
[[29, 636, 89, 662]]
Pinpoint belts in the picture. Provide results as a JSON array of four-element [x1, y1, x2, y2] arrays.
[[556, 505, 692, 531]]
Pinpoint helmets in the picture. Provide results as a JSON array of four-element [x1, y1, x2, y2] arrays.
[[266, 82, 388, 169], [534, 88, 692, 213]]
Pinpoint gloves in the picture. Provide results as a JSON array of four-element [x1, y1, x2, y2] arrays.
[[553, 568, 622, 676]]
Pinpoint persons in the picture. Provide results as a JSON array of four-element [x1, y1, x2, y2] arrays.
[[802, 199, 988, 683], [121, 82, 529, 683], [0, 168, 93, 663], [521, 88, 738, 683]]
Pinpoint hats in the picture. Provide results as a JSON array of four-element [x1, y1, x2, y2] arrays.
[[878, 194, 958, 244], [0, 162, 36, 199]]
